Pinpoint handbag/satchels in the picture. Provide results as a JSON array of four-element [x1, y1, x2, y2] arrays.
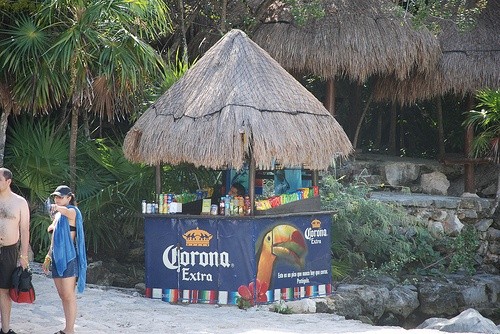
[[10, 266, 35, 303]]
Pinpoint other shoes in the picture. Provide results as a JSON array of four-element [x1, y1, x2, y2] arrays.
[[54, 330, 65, 334]]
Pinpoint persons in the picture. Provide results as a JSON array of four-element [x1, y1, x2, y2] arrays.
[[228, 182, 246, 198], [42, 186, 87, 334], [0, 168, 30, 334]]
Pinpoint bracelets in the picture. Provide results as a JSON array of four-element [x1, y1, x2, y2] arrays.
[[19, 256, 29, 263], [45, 255, 52, 261]]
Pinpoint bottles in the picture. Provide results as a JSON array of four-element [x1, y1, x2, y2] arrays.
[[142, 185, 275, 216]]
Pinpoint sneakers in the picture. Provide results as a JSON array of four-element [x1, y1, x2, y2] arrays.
[[0, 328, 17, 334]]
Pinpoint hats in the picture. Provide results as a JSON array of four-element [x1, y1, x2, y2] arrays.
[[51, 185, 72, 197]]
